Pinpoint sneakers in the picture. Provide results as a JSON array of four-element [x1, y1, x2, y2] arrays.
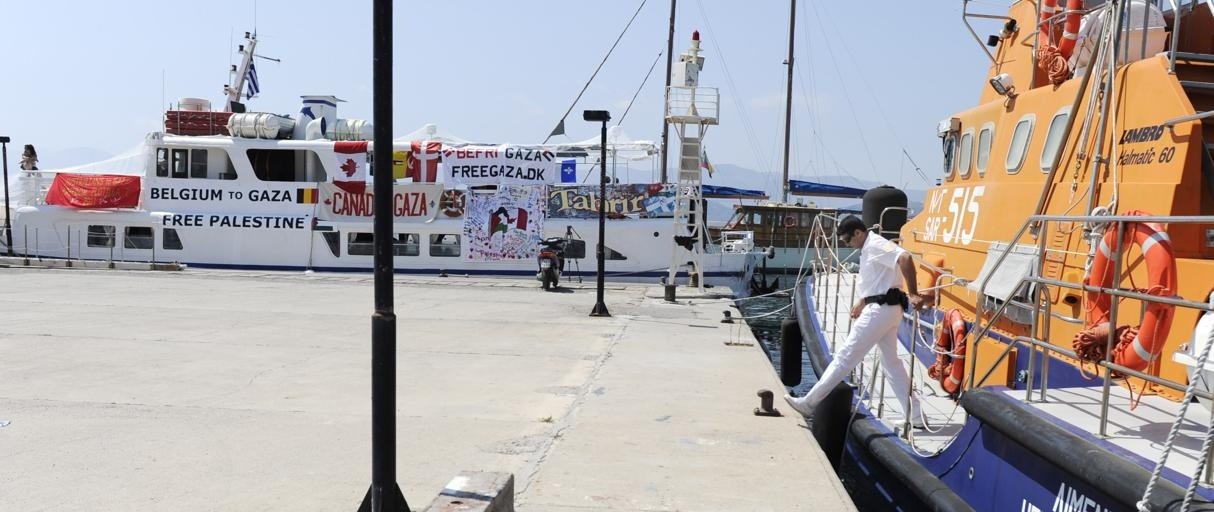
[[909, 415, 929, 429], [784, 394, 816, 419]]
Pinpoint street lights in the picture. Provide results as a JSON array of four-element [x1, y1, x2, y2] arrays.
[[0, 136, 13, 257], [584, 108, 611, 317]]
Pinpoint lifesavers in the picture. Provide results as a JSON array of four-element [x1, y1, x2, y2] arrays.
[[935, 308, 966, 395], [784, 216, 796, 228], [440, 190, 465, 217], [1084, 208, 1178, 379], [1038, 0, 1082, 61]]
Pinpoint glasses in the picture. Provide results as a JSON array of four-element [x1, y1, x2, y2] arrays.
[[842, 232, 853, 245]]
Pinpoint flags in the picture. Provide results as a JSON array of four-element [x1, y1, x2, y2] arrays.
[[411, 142, 439, 183], [243, 58, 259, 101], [330, 140, 367, 197], [700, 150, 714, 179]]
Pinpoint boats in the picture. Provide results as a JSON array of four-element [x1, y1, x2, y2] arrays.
[[707, 0, 861, 275], [794, 0, 1214, 512], [0, 32, 754, 273]]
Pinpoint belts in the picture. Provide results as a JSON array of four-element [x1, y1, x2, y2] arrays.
[[863, 293, 888, 305]]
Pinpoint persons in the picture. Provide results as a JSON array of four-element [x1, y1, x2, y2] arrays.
[[783, 214, 930, 430]]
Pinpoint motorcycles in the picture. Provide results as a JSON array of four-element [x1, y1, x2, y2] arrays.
[[537, 237, 568, 289]]
